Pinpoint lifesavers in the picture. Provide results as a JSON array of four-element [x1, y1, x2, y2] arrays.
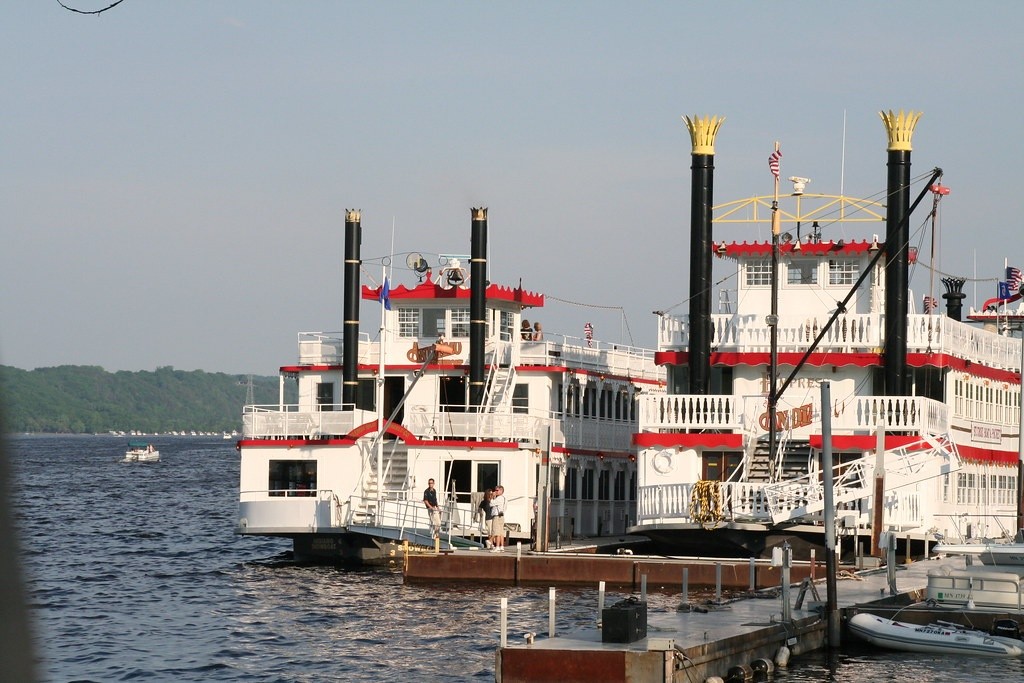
[[653, 450, 676, 474]]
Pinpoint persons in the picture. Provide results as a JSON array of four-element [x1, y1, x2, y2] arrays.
[[478, 485, 508, 553], [422, 478, 442, 540], [520, 320, 544, 341]]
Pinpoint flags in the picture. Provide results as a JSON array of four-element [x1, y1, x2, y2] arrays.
[[923, 296, 938, 314], [1006, 266, 1023, 291], [768, 149, 783, 181]]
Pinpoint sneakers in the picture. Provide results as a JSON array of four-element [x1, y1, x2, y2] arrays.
[[490, 546, 505, 553], [485, 539, 496, 550]]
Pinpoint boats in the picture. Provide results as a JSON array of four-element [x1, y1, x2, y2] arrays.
[[927, 514, 1024, 615], [233, 206, 667, 558], [126, 449, 160, 463], [848, 613, 1024, 657], [626, 108, 1024, 557], [129, 441, 148, 447]]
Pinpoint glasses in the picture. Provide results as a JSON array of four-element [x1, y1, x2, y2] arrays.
[[429, 483, 433, 484], [495, 490, 498, 491]]
